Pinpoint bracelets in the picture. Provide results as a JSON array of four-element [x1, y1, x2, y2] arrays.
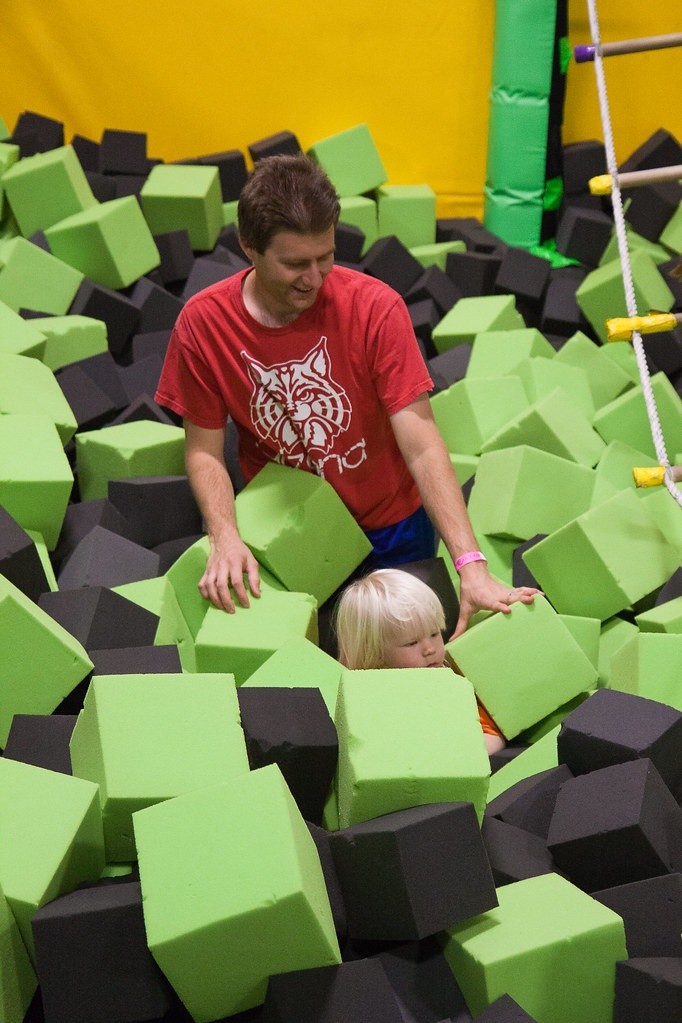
[[453, 551, 488, 570]]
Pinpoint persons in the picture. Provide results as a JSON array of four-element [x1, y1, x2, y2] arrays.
[[333, 567, 506, 758], [157, 156, 539, 641]]
